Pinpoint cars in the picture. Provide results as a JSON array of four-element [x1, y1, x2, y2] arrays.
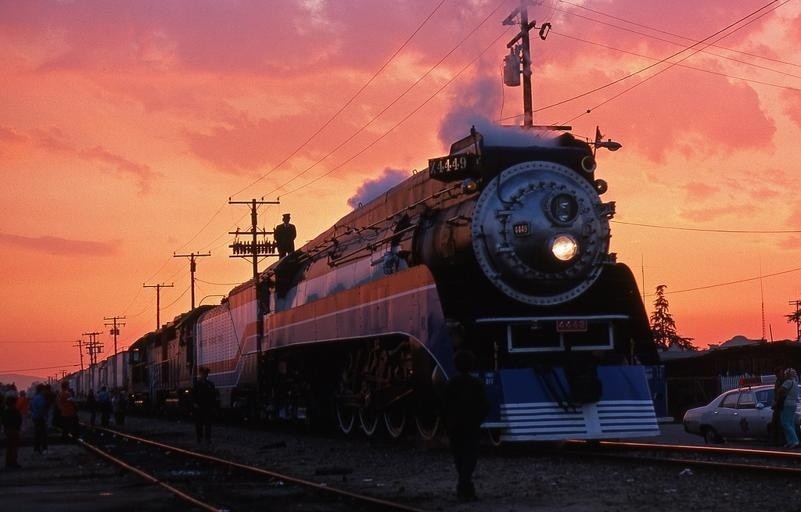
[[683, 373, 801, 445]]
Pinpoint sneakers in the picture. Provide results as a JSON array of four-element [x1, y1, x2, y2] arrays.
[[195, 437, 212, 445], [5, 448, 48, 472]]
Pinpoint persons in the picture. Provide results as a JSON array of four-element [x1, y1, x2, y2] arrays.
[[274, 213, 298, 261], [734, 367, 756, 387], [191, 366, 219, 446], [0, 380, 130, 474], [437, 354, 490, 501], [777, 367, 801, 449], [769, 367, 789, 447]]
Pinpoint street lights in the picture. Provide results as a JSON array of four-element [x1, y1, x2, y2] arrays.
[[508, 17, 552, 127]]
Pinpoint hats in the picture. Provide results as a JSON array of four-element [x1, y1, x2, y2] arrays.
[[199, 367, 211, 372], [282, 213, 291, 217]]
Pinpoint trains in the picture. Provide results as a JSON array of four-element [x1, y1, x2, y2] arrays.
[[27, 121, 661, 445]]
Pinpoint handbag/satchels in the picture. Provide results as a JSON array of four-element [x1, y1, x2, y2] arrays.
[[51, 413, 80, 429]]
